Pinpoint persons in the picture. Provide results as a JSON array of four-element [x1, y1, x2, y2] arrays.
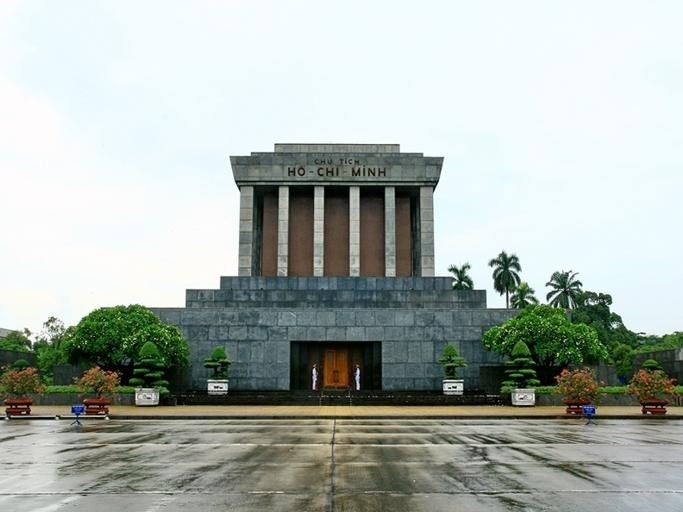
[[312, 363, 318, 390], [353, 364, 361, 391]]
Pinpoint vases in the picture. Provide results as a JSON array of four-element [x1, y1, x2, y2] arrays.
[[3, 398, 32, 416], [561, 398, 591, 414], [640, 398, 667, 415], [83, 397, 109, 413]]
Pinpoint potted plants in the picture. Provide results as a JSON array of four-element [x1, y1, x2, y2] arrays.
[[439, 345, 465, 395], [499, 337, 540, 406], [129, 341, 169, 404], [202, 343, 231, 394]]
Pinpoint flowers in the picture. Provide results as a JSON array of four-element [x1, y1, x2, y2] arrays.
[[624, 369, 677, 401], [554, 365, 609, 408], [0, 368, 49, 404], [69, 364, 120, 399]]
